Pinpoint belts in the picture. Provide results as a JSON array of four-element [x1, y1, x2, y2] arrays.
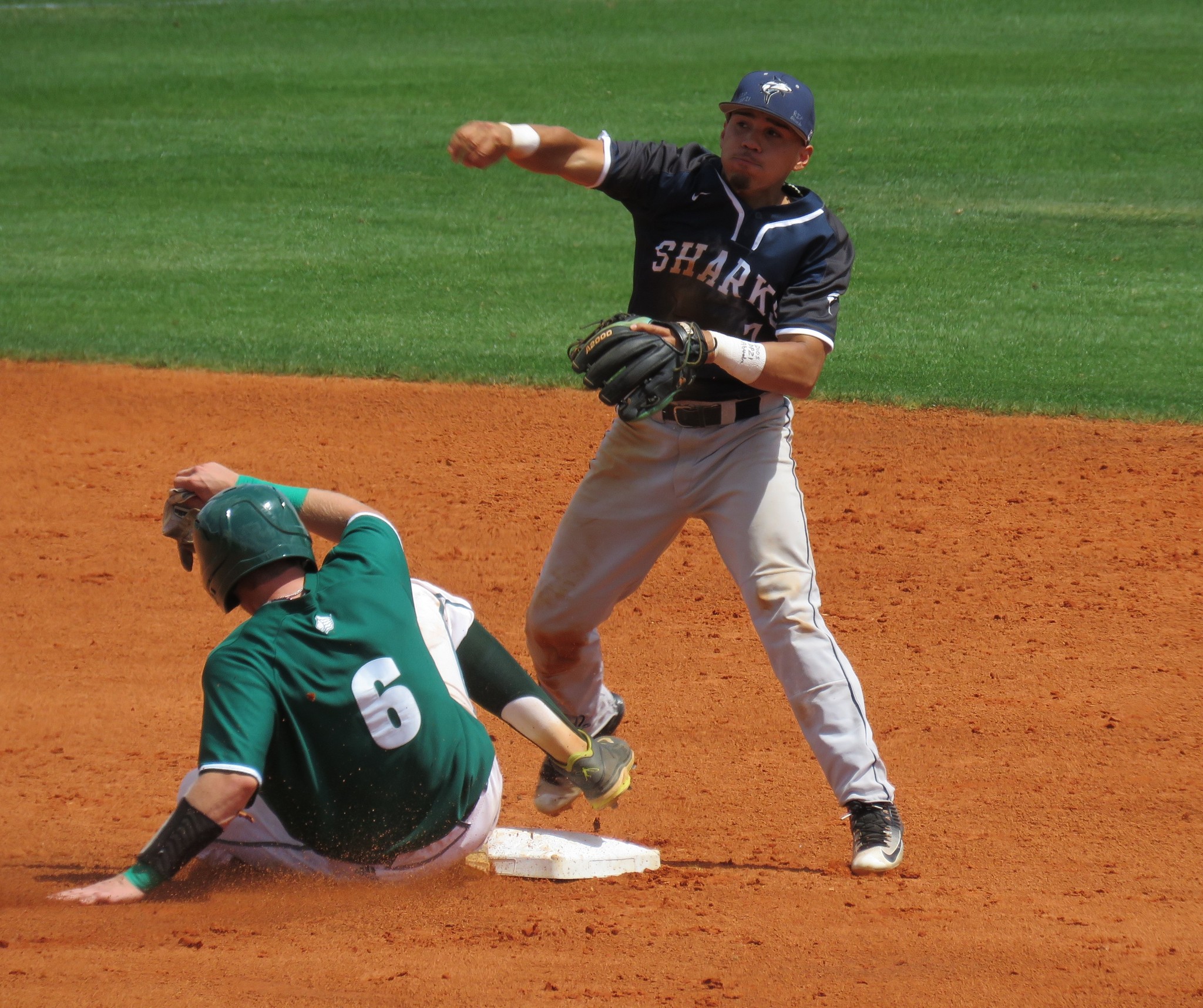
[[662, 401, 758, 429]]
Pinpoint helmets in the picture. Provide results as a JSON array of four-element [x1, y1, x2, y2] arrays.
[[194, 482, 319, 615]]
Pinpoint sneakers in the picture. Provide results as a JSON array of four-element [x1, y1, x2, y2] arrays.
[[533, 691, 626, 818], [844, 799, 904, 872], [549, 723, 633, 809]]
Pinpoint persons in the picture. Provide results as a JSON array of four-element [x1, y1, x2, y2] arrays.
[[450, 70, 904, 877], [47, 461, 634, 906]]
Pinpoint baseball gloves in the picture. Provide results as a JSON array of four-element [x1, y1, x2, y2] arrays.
[[572, 313, 710, 424]]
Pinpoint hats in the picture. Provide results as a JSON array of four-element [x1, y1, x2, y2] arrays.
[[718, 71, 814, 145]]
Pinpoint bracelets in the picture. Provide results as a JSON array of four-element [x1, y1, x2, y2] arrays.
[[498, 121, 542, 161], [708, 331, 766, 385]]
